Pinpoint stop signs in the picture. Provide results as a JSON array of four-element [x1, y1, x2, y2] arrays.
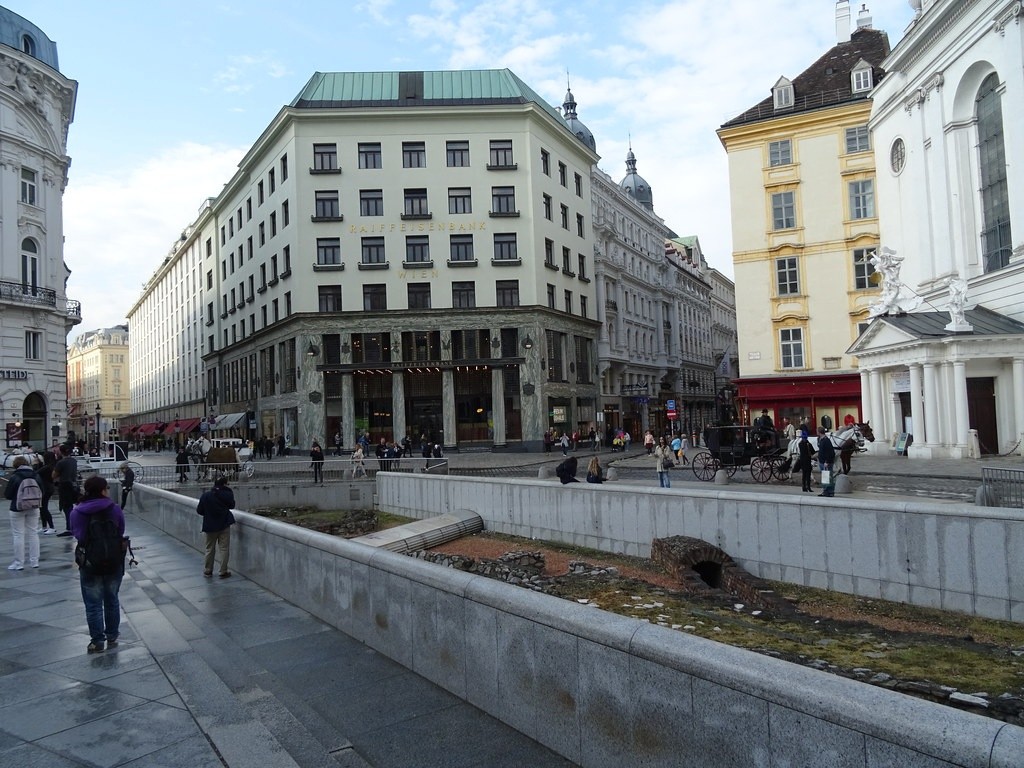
[[667, 410, 677, 420]]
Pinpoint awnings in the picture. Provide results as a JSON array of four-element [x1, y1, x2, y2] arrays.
[[161, 418, 201, 433], [119, 423, 136, 435], [208, 412, 247, 430], [133, 422, 164, 436]]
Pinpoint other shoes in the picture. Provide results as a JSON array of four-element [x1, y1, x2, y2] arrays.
[[203, 570, 213, 578], [219, 570, 231, 578], [88, 641, 105, 652], [802, 487, 814, 493], [44, 528, 58, 535], [56, 532, 72, 536], [32, 562, 39, 568], [818, 492, 834, 497], [107, 639, 118, 649], [38, 528, 48, 534], [687, 461, 690, 466], [8, 563, 24, 570]]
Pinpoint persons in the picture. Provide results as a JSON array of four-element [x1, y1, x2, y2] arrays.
[[611, 427, 631, 453], [196, 477, 236, 579], [868, 252, 969, 327], [4, 456, 42, 571], [117, 462, 134, 510], [69, 474, 126, 654], [758, 408, 835, 497], [403, 433, 414, 458], [331, 431, 344, 458], [138, 436, 180, 452], [543, 429, 581, 457], [586, 456, 608, 485], [176, 446, 190, 483], [555, 455, 581, 485], [587, 427, 604, 453], [351, 431, 370, 478], [420, 433, 443, 471], [375, 437, 403, 472], [34, 444, 77, 535], [12, 437, 104, 462], [245, 433, 291, 460], [309, 438, 324, 487], [643, 430, 690, 488]]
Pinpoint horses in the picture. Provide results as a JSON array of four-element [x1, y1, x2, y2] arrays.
[[0, 448, 45, 471], [810, 420, 877, 474], [185, 436, 241, 480], [777, 424, 864, 484]]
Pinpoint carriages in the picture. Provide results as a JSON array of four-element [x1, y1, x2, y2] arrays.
[[0, 441, 144, 483], [185, 435, 255, 482], [692, 420, 875, 484]]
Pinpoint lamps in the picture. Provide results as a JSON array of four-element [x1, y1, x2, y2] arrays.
[[11, 412, 22, 426], [523, 332, 532, 350], [55, 414, 62, 426], [308, 340, 321, 357]]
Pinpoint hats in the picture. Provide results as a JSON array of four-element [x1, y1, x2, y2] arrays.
[[761, 408, 770, 413]]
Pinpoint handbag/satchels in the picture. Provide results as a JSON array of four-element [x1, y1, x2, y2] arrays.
[[822, 466, 831, 485], [678, 448, 685, 457], [663, 456, 674, 468]]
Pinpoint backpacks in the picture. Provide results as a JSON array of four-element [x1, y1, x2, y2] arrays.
[[15, 472, 42, 510], [78, 503, 122, 575]]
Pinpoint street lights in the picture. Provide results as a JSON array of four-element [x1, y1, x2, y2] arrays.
[[84, 411, 88, 454], [96, 403, 102, 457], [155, 418, 159, 453], [175, 413, 179, 450], [209, 407, 215, 439]]
